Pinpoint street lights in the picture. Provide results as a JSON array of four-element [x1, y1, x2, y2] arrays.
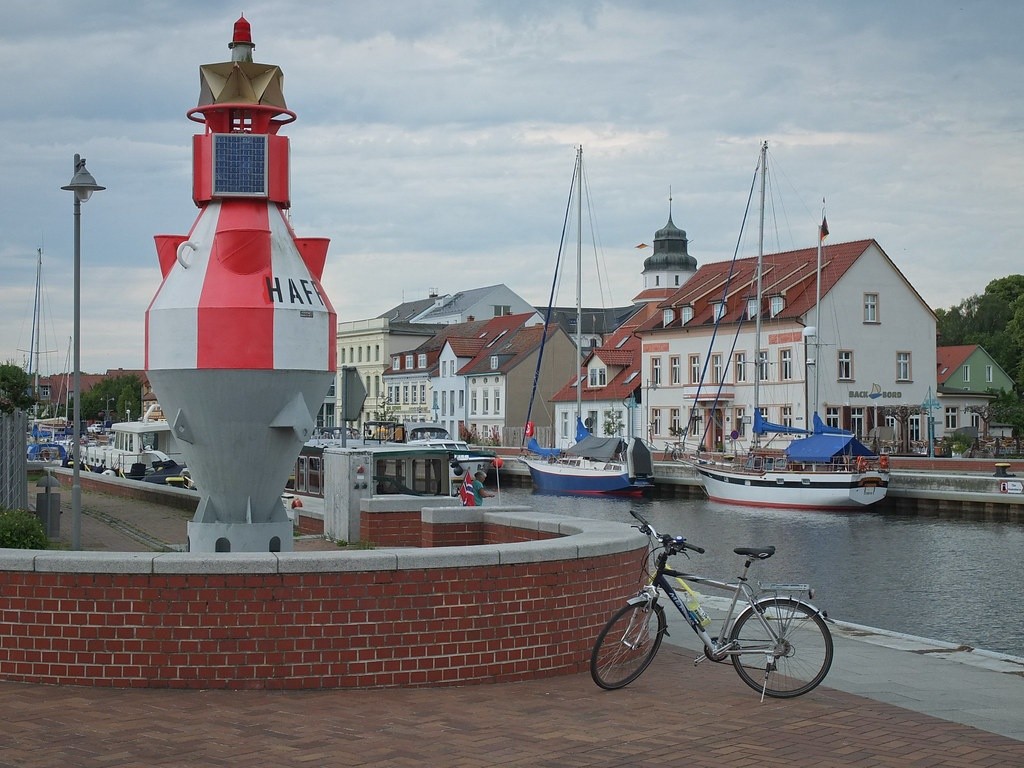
[[60, 153, 106, 550], [100, 396, 114, 419], [921, 387, 941, 458], [627, 388, 639, 437]]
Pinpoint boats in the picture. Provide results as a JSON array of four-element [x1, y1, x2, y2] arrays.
[[385, 423, 496, 484], [69, 404, 196, 488]]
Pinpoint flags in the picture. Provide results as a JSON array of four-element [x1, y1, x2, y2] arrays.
[[459, 471, 475, 506], [820, 211, 829, 241], [634, 243, 648, 249]]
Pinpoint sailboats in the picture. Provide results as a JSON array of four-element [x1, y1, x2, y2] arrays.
[[675, 140, 890, 512], [517, 144, 654, 498], [16, 233, 67, 473]]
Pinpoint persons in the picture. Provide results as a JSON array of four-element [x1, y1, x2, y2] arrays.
[[472, 470, 495, 506]]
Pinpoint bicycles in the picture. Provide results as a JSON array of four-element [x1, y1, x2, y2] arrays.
[[590, 511, 835, 703], [662, 441, 684, 462]]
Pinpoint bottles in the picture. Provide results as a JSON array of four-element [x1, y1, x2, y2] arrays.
[[684, 591, 712, 627]]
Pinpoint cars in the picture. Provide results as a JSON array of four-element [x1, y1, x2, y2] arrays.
[[87, 424, 101, 434]]
